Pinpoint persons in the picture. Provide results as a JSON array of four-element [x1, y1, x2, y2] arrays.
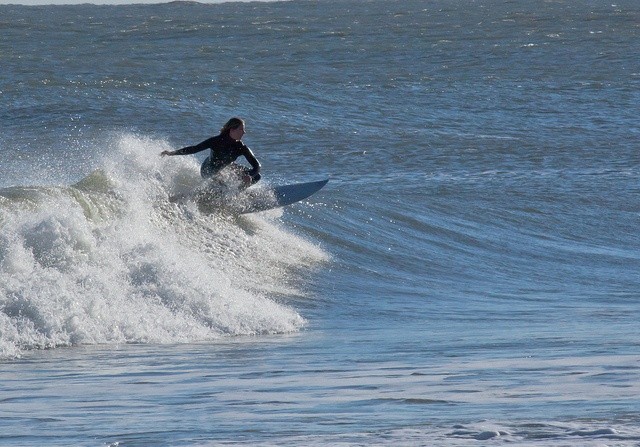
[[158, 118, 261, 185]]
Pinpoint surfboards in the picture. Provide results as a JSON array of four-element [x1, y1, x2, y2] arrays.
[[168, 178, 329, 222]]
